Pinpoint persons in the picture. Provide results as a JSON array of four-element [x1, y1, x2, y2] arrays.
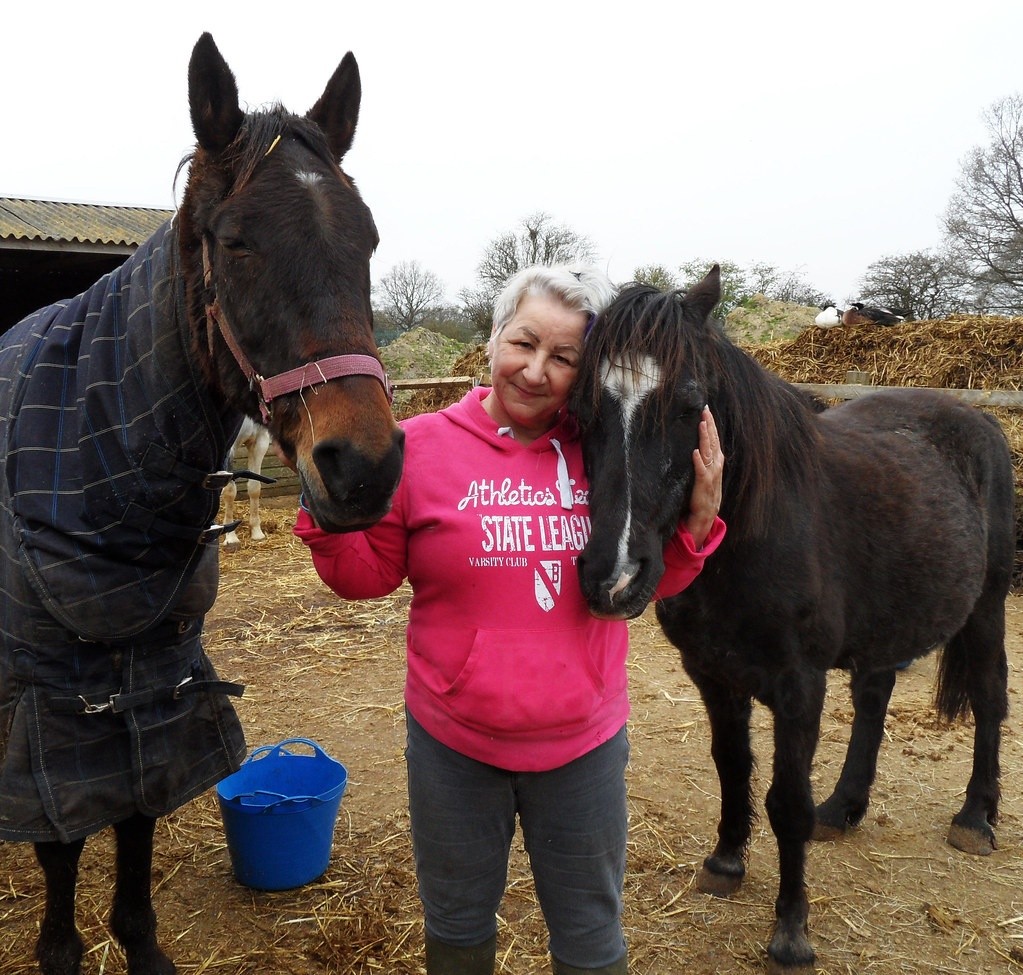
[[270, 261, 726, 975]]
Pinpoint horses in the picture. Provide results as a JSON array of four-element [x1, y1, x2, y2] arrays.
[[565, 261, 1018, 975], [2, 30, 408, 975]]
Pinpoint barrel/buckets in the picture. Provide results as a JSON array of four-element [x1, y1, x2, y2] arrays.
[[216, 737, 347, 813], [216, 746, 348, 891]]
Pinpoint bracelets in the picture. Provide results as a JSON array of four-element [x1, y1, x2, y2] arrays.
[[299, 492, 310, 515]]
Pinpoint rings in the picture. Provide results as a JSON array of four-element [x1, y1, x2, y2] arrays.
[[704, 459, 714, 466]]
